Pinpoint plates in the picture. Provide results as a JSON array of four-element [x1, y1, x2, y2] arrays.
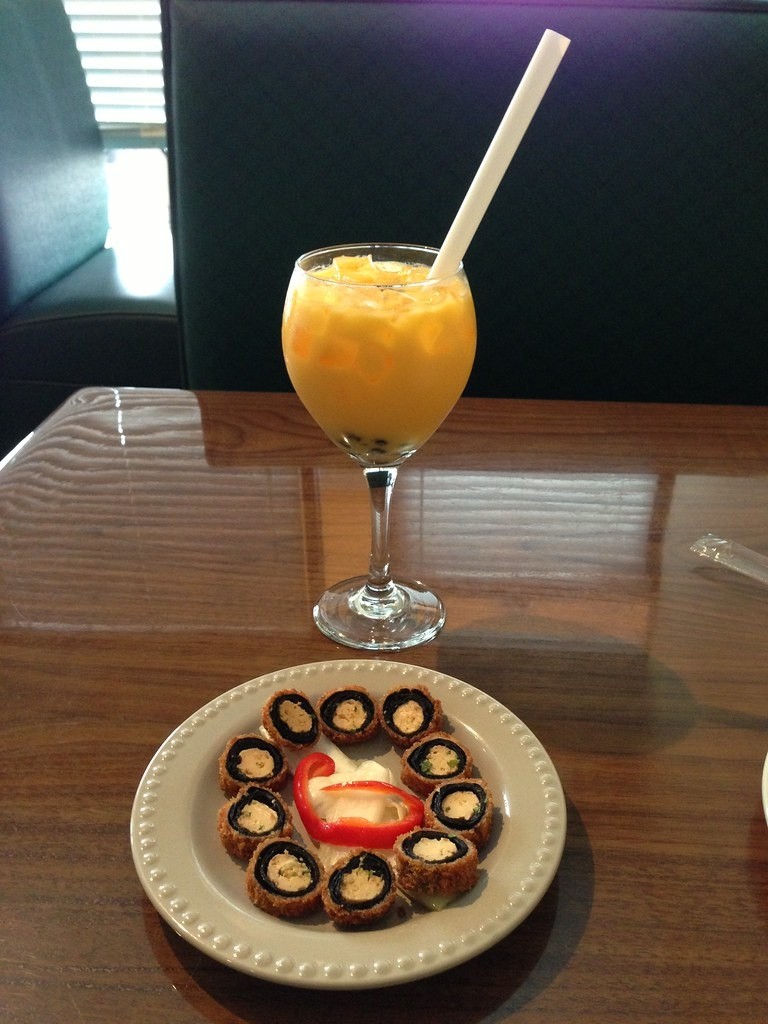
[[129, 659, 568, 989]]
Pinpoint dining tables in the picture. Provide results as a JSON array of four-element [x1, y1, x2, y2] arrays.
[[1, 384, 768, 1024]]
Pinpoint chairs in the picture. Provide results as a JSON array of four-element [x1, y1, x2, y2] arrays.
[[0, 0, 193, 452], [159, 2, 767, 406]]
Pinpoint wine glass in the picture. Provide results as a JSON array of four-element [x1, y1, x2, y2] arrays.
[[283, 242, 477, 650]]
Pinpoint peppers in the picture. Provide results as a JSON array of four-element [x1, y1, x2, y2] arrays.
[[294, 751, 424, 849]]
